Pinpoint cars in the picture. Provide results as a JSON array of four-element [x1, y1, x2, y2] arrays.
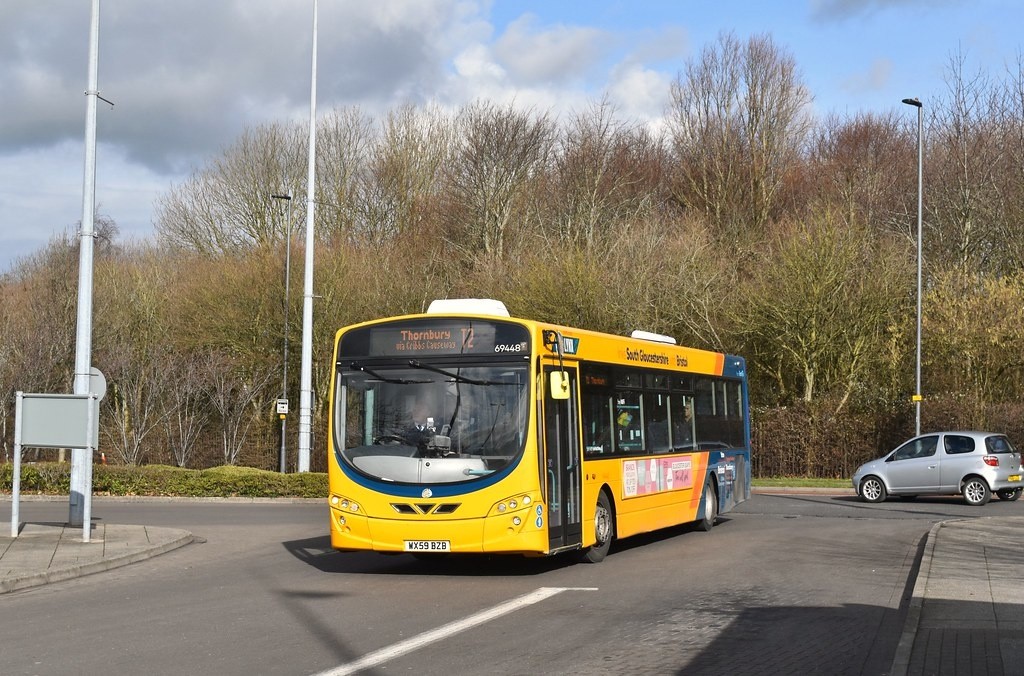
[[853, 430, 1024, 506]]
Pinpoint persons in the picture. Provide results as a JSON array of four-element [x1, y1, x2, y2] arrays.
[[682, 401, 693, 442], [385, 397, 441, 459]]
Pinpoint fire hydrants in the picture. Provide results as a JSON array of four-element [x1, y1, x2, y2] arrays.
[[100, 453, 106, 465]]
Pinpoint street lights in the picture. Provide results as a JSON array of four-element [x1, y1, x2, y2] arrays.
[[270, 194, 292, 471], [904, 98, 922, 454]]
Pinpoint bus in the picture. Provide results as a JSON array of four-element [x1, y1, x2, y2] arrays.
[[326, 297, 752, 564]]
